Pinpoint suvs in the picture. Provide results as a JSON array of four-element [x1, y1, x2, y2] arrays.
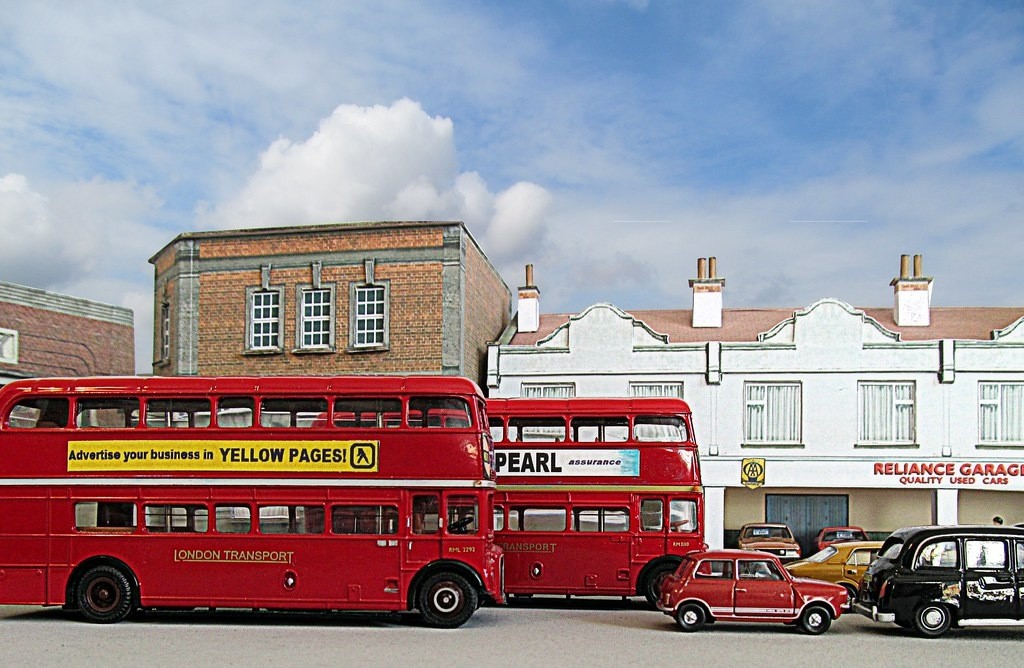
[[852, 522, 1024, 640]]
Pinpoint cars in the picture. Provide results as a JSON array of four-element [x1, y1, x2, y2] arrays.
[[737, 523, 802, 573], [814, 525, 872, 555], [782, 541, 924, 614], [654, 548, 853, 636]]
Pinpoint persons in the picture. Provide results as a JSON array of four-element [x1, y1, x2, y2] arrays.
[[993, 517, 1003, 526], [37, 402, 66, 428], [414, 497, 434, 533]]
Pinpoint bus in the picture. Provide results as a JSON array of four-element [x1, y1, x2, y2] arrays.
[[1, 370, 509, 629], [305, 396, 712, 608]]
